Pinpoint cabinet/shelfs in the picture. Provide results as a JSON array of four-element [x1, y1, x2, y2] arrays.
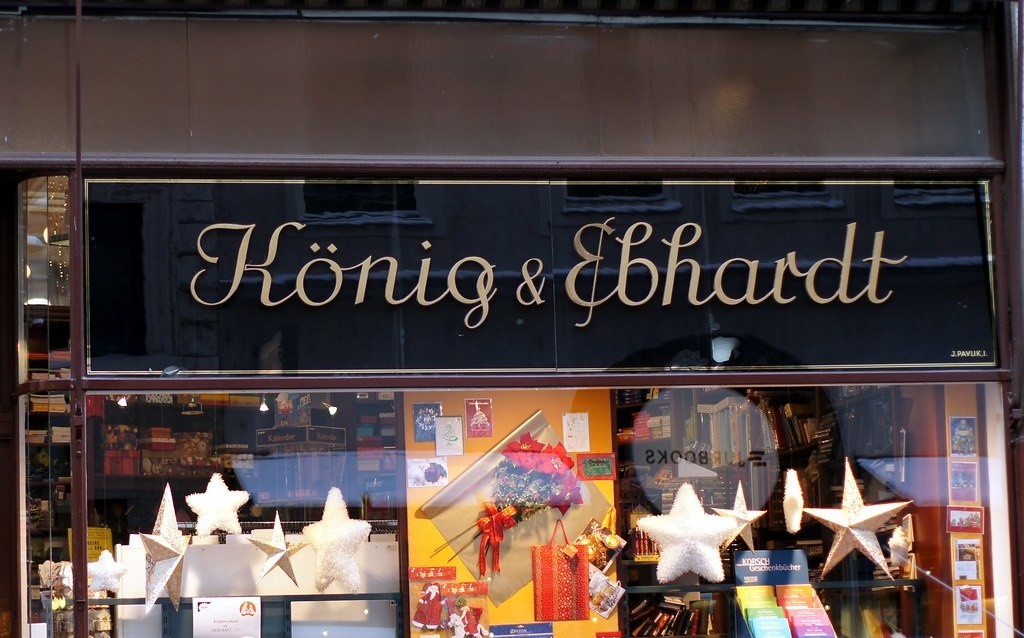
[[331, 393, 397, 519], [88, 394, 276, 544], [610, 388, 920, 638], [25, 305, 72, 624]]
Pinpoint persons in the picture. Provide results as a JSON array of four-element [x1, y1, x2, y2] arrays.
[[460, 606, 478, 638], [953, 419, 973, 456], [952, 512, 980, 528]]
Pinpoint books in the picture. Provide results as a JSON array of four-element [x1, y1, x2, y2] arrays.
[[624, 397, 918, 637], [28, 350, 72, 413], [736, 584, 835, 638]]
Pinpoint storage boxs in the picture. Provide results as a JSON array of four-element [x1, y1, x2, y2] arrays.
[[99, 422, 258, 480], [357, 414, 397, 520]]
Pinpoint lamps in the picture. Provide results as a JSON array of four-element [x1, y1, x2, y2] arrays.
[[181, 395, 205, 415], [258, 395, 269, 413], [319, 400, 338, 415]]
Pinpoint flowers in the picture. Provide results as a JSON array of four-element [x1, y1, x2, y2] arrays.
[[431, 432, 586, 568]]
